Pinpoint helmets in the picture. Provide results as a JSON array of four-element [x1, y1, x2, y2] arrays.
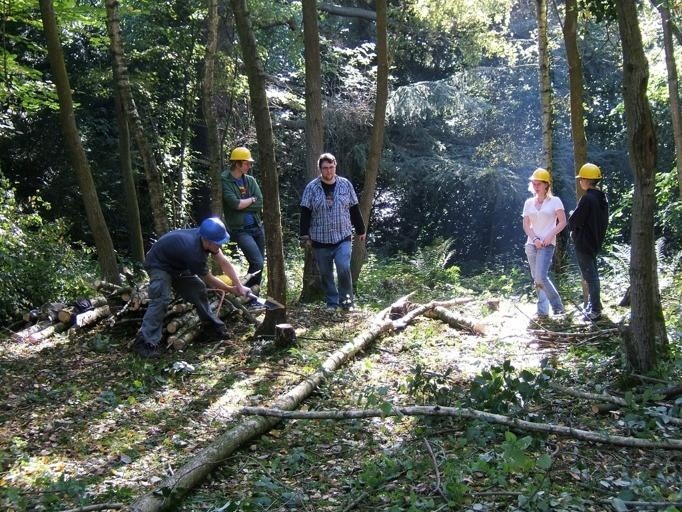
[[528, 168, 550, 183], [575, 163, 602, 179], [199, 218, 230, 245], [229, 147, 254, 161]]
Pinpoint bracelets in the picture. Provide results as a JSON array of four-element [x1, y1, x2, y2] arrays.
[[531, 237, 539, 243]]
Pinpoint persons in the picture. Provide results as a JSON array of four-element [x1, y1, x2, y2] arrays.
[[134, 216, 252, 355], [299, 154, 368, 315], [522, 168, 567, 322], [220, 146, 266, 290], [566, 162, 609, 322]]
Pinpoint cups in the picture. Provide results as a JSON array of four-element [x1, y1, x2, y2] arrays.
[[275, 324, 294, 346]]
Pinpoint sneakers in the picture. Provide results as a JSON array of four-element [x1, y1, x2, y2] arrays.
[[132, 327, 161, 351], [582, 311, 602, 321]]
